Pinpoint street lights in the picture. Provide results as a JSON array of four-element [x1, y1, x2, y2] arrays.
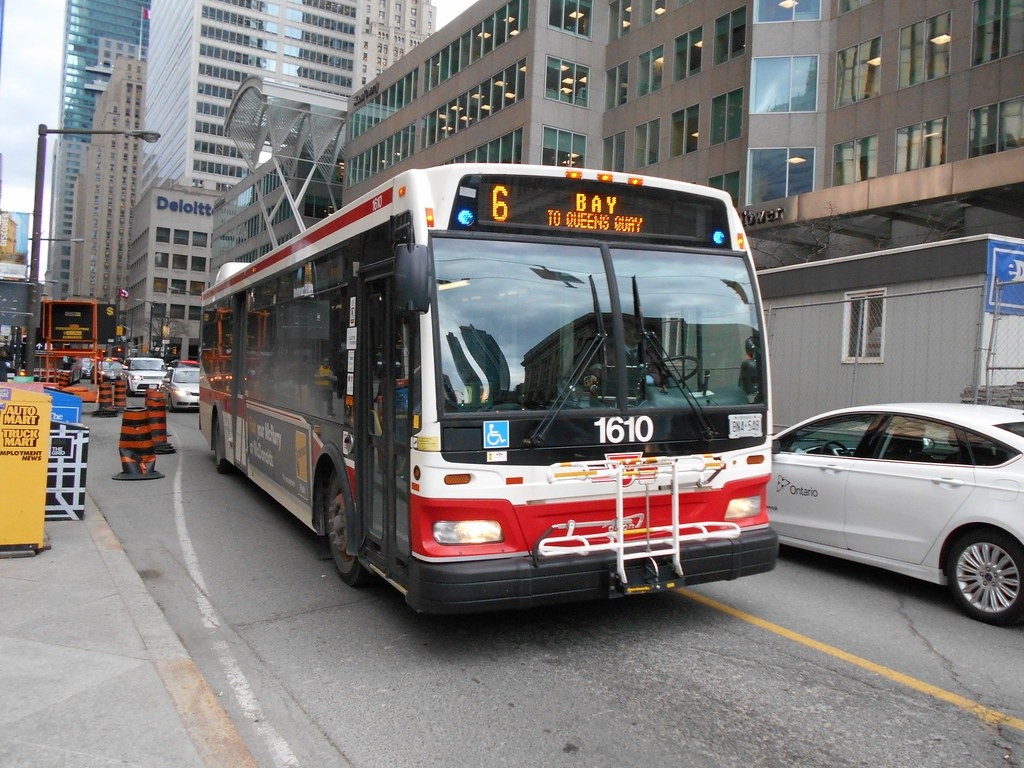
[[168, 287, 201, 295], [15, 238, 85, 375], [136, 299, 154, 351], [26, 124, 161, 376]]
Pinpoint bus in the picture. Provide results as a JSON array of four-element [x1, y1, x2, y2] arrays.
[[199, 162, 779, 615]]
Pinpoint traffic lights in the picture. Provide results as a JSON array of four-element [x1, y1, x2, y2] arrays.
[[117, 347, 122, 352], [35, 338, 45, 350]]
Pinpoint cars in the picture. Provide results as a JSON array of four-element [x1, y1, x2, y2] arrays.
[[19, 355, 82, 385], [81, 358, 94, 379], [766, 404, 1024, 623], [167, 360, 200, 370], [159, 368, 201, 412], [90, 361, 128, 384], [120, 358, 173, 397]]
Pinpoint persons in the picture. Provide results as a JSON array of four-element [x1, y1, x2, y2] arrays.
[[739, 338, 758, 395]]
